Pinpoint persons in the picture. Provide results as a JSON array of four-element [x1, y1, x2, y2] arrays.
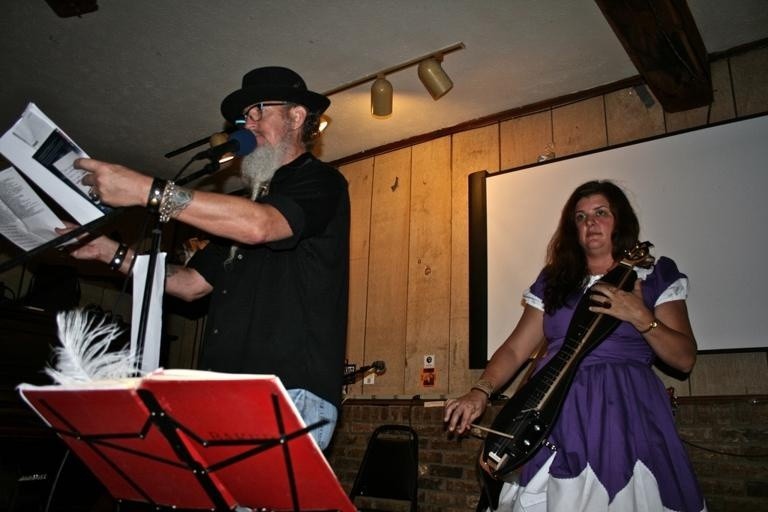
[[54, 65, 351, 451], [442, 178, 708, 511]]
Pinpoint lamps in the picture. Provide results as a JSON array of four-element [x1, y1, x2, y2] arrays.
[[163, 40, 465, 162]]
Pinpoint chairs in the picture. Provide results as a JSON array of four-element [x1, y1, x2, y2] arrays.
[[349, 423, 421, 511]]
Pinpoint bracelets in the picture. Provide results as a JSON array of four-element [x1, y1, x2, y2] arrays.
[[637, 316, 658, 336], [469, 384, 490, 399], [126, 246, 142, 277], [107, 241, 130, 273], [146, 176, 167, 215], [160, 179, 177, 224]]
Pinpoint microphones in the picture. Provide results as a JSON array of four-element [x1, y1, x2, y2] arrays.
[[191, 128, 257, 160]]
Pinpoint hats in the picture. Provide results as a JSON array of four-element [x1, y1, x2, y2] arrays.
[[220, 66, 331, 125]]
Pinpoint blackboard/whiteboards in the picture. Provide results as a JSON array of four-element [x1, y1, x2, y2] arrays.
[[468, 111, 768, 370]]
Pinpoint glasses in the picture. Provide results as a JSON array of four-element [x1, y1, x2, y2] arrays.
[[234, 102, 288, 127]]
